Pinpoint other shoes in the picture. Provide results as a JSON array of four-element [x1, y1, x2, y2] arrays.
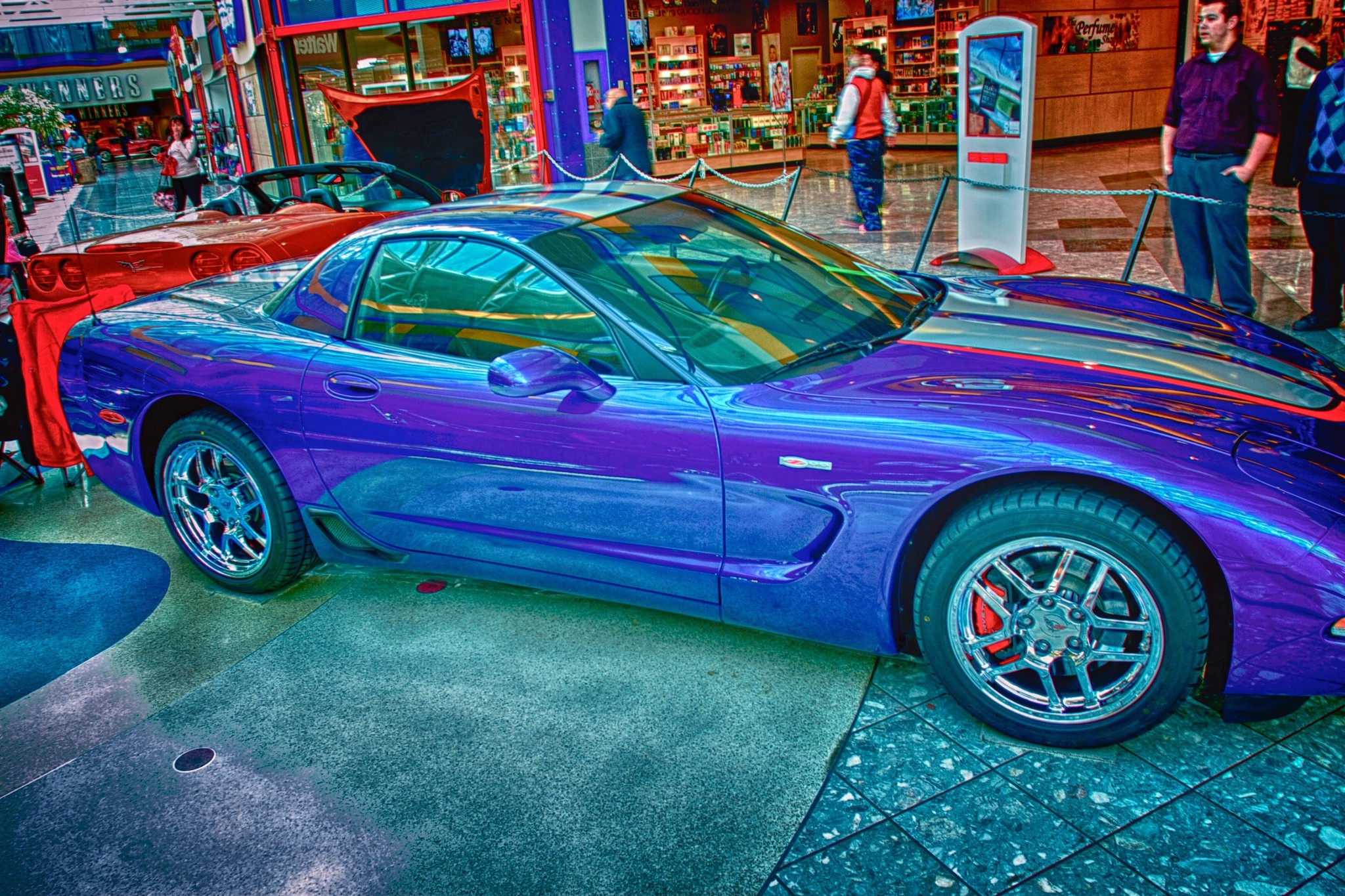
[[840, 218, 861, 227], [859, 224, 881, 231], [1293, 312, 1342, 331]]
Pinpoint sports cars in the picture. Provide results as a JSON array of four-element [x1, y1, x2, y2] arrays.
[[0, 63, 495, 299], [90, 136, 169, 163], [57, 179, 1343, 749]]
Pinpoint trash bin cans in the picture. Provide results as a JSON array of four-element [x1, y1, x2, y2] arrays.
[[344, 129, 392, 201]]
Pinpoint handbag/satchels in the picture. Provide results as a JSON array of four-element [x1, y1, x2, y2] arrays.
[[152, 185, 178, 212], [842, 124, 857, 140]]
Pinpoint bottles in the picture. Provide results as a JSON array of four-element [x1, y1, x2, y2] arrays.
[[485, 82, 538, 161]]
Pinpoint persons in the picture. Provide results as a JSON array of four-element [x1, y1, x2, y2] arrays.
[[1272, 24, 1328, 186], [1290, 58, 1345, 331], [798, 5, 817, 35], [739, 77, 760, 102], [5, 217, 31, 300], [344, 125, 392, 201], [65, 127, 131, 174], [592, 88, 652, 182], [1042, 16, 1082, 56], [160, 115, 204, 219], [827, 48, 898, 232], [769, 44, 788, 109], [1159, 0, 1279, 319]]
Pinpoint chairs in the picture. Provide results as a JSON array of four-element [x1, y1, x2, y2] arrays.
[[205, 198, 244, 215], [303, 188, 343, 213]]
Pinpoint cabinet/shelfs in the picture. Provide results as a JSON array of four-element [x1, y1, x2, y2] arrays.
[[298, 46, 541, 202], [640, 108, 807, 178], [191, 109, 240, 182], [800, 97, 959, 151], [843, 5, 979, 96], [630, 35, 762, 110]]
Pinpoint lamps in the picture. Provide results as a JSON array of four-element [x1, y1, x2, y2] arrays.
[[101, 7, 115, 30], [117, 22, 129, 53]]
